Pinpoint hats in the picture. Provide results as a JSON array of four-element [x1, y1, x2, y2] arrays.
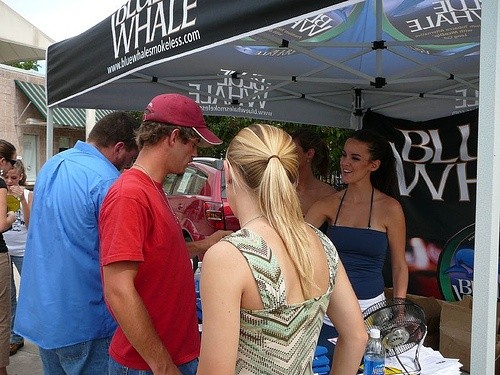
[[142, 93, 223, 145]]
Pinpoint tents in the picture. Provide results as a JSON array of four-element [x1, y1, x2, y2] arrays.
[[46, 0, 500, 375]]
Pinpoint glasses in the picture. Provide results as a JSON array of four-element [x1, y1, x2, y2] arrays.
[[6, 158, 16, 166]]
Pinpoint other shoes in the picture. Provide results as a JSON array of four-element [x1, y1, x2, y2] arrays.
[[9, 339, 24, 355]]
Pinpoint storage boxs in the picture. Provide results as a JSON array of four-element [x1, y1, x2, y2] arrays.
[[384, 288, 446, 350], [439, 296, 500, 372]]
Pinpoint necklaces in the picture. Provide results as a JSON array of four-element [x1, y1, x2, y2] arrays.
[[240, 215, 265, 228], [133, 163, 179, 225]]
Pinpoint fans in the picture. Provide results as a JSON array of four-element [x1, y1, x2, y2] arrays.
[[362, 297, 429, 374]]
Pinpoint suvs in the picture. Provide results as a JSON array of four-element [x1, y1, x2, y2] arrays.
[[164, 156, 241, 273]]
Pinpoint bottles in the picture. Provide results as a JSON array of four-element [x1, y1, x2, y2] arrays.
[[11, 212, 21, 232], [364, 329, 386, 374], [194, 262, 203, 324]]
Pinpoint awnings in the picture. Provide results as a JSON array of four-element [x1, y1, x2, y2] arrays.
[[0, 0, 57, 64], [15, 80, 118, 127]]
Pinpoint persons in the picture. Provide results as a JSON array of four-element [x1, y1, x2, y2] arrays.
[[0, 94, 408, 375]]
